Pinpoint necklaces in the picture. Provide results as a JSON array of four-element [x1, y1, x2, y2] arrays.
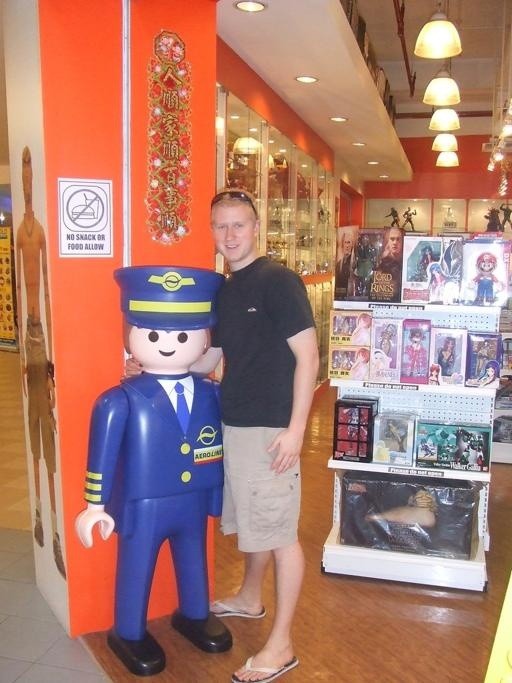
[[23, 210, 34, 237]]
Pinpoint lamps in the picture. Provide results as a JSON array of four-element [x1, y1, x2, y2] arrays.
[[436, 152, 459, 170], [431, 132, 461, 151], [423, 60, 461, 107], [429, 108, 461, 132], [413, 0, 465, 60]]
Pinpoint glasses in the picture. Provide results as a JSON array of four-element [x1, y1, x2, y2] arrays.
[[211, 191, 257, 215]]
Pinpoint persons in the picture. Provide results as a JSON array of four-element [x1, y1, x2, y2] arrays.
[[338, 228, 355, 286], [77, 263, 232, 675], [380, 227, 402, 275], [403, 206, 416, 231], [500, 203, 512, 231], [15, 143, 65, 581], [125, 191, 322, 681], [384, 207, 401, 226]]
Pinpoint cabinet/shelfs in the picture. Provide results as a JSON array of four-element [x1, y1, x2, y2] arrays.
[[215, 80, 336, 410], [365, 197, 512, 232], [319, 220, 511, 593]]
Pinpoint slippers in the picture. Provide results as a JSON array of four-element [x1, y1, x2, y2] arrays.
[[209, 600, 266, 618], [231, 656, 299, 683]]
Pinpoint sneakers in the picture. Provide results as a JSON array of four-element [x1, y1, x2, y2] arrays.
[[53, 533, 66, 581], [35, 522, 44, 547]]
